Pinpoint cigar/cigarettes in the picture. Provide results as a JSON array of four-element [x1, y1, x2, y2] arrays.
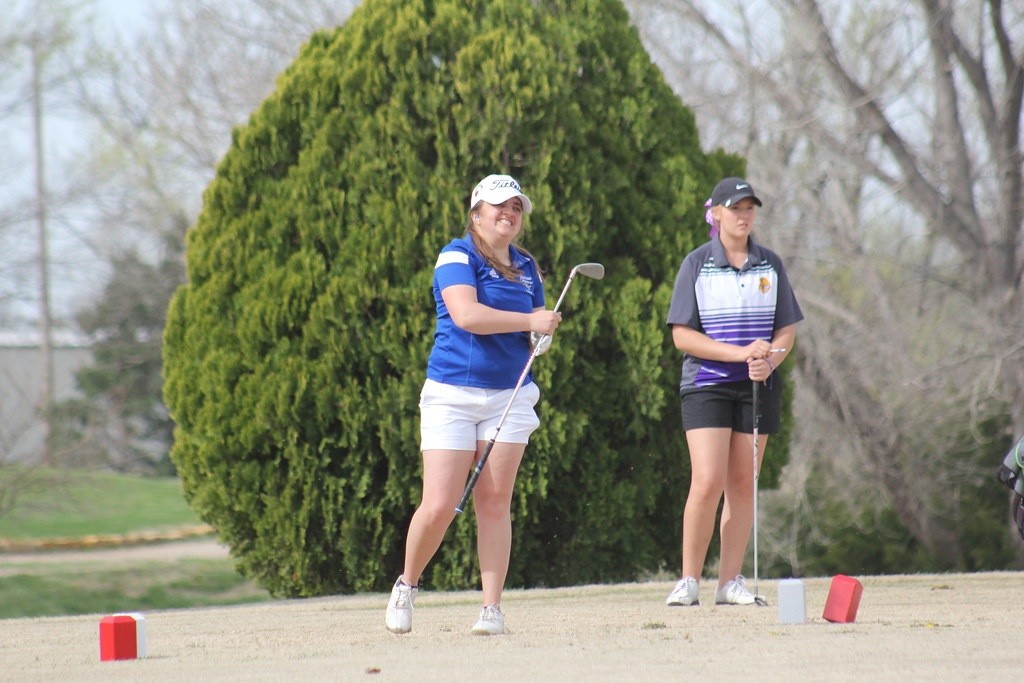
[[771, 349, 787, 352]]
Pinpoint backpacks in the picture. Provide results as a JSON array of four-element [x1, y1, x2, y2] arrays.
[[996, 431, 1023, 539]]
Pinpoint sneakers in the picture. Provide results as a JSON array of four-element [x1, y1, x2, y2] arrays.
[[714, 579, 766, 604], [665, 577, 700, 606], [385, 573, 418, 633], [472, 605, 505, 636]]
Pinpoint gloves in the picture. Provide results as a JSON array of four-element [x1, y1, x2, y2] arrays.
[[530, 331, 553, 356]]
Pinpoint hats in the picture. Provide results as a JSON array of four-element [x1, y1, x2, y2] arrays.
[[470, 173, 533, 215], [710, 177, 763, 209]]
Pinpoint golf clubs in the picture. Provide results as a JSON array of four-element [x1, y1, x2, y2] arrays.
[[455, 262, 605, 515], [753, 380, 769, 607]]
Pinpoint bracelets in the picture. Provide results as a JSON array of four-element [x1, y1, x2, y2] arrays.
[[763, 358, 774, 374]]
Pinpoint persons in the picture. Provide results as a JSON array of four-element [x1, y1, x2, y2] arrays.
[[665, 177, 804, 607], [386, 173, 563, 636]]
